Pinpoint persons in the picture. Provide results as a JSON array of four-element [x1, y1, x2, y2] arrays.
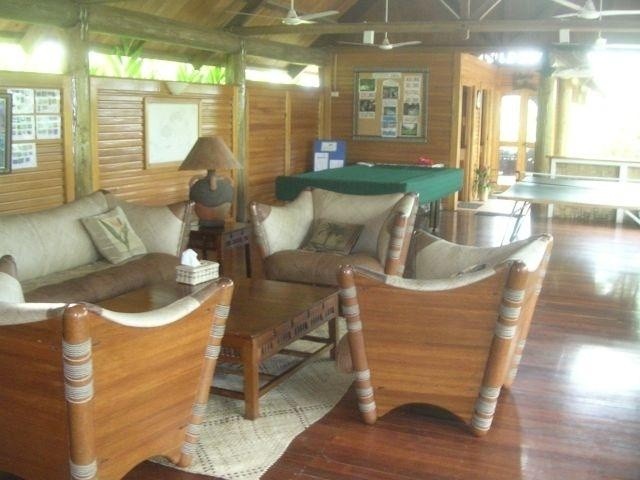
[[360, 99, 375, 111]]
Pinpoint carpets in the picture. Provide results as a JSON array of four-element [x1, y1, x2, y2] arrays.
[[146, 313, 357, 479]]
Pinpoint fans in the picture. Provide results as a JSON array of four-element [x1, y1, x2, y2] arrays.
[[552, 0, 640, 20], [335, 36, 421, 51], [224, 8, 341, 26]]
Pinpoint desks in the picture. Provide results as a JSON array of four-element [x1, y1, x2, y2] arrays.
[[498, 170, 640, 244], [275, 161, 464, 231]]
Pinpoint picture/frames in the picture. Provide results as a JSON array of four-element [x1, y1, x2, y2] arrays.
[[0, 92, 12, 176], [143, 97, 203, 171]]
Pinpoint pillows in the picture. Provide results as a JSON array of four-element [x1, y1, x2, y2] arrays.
[[302, 218, 365, 258], [80, 206, 146, 264], [450, 262, 489, 278]]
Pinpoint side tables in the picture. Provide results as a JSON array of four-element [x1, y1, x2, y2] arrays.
[[188, 221, 252, 279]]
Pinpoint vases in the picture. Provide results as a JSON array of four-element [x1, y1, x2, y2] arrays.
[[479, 187, 491, 201]]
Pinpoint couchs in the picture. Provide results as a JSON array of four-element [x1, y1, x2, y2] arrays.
[[0, 186, 196, 303]]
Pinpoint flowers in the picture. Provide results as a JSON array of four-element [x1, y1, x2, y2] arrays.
[[473, 164, 497, 193]]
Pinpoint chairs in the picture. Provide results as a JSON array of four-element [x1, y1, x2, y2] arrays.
[[247, 187, 419, 288], [0, 256, 234, 480], [337, 230, 552, 435]]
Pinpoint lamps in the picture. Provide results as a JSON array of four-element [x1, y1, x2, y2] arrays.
[[178, 136, 245, 225]]
